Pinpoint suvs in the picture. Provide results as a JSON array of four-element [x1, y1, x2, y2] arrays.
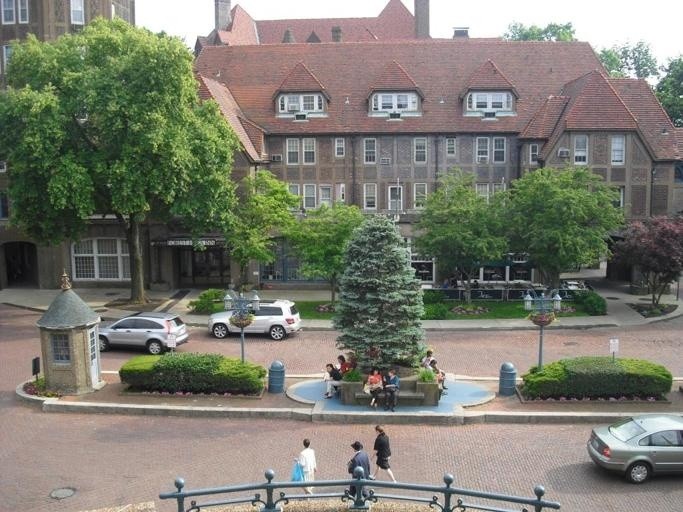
[[208, 299, 301, 341], [97, 312, 189, 355]]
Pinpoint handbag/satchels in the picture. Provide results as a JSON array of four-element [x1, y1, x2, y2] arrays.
[[293, 462, 303, 481]]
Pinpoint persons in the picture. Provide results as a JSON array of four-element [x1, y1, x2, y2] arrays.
[[323, 348, 449, 412], [369, 424, 397, 483], [347, 440, 371, 501], [440, 279, 594, 300], [293, 438, 317, 495]]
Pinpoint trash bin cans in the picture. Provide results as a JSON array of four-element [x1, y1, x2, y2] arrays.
[[267, 360, 285, 394], [499, 362, 517, 396]]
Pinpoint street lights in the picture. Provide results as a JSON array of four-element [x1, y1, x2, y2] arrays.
[[224, 290, 260, 363], [523, 288, 563, 372]]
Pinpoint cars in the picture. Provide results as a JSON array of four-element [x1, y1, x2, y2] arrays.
[[586, 414, 682, 485]]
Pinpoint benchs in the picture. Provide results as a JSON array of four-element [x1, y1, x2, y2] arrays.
[[355, 390, 425, 406], [437, 379, 443, 401]]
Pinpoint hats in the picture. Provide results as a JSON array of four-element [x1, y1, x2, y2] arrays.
[[351, 441, 362, 450]]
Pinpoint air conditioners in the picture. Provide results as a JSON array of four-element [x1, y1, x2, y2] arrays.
[[288, 104, 300, 110], [380, 158, 390, 165], [272, 154, 281, 161]]
